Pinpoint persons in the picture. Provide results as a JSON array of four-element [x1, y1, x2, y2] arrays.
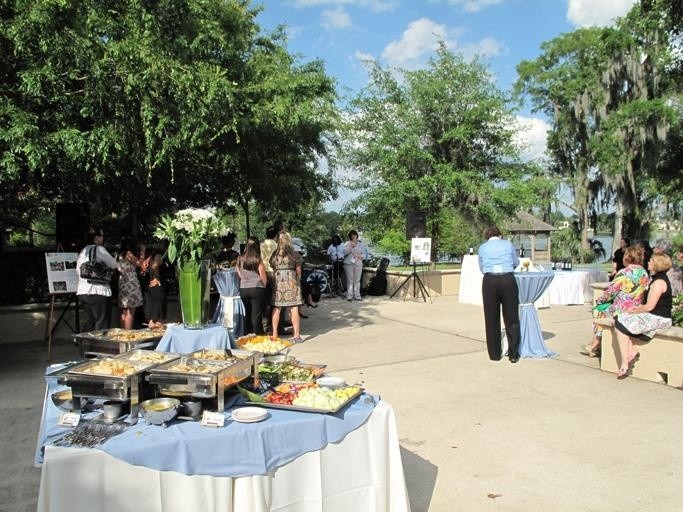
[[118, 239, 141, 330], [478, 227, 520, 363], [217, 221, 318, 342], [327, 234, 345, 287], [142, 246, 167, 323], [581, 237, 672, 379], [340, 230, 368, 301], [75, 231, 117, 330]]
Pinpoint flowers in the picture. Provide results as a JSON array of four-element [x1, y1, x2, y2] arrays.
[[152, 206, 233, 258]]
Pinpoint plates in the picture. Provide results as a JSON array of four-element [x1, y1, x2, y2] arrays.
[[231, 407, 268, 423], [315, 377, 344, 386]]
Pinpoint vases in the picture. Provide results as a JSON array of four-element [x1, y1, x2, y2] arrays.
[[177, 261, 209, 329]]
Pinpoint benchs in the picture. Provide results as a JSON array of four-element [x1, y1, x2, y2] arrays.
[[593, 318, 683, 388]]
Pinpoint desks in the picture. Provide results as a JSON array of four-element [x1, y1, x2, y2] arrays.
[[534, 269, 589, 308], [211, 269, 247, 344], [458, 254, 531, 307], [514, 271, 560, 359]]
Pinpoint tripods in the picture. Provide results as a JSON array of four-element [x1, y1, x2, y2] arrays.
[[390, 262, 430, 304]]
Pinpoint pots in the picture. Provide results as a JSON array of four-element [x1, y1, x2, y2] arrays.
[[52, 390, 88, 412], [139, 397, 181, 428]]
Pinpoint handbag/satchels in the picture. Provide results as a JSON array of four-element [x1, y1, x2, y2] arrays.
[[80, 245, 115, 283]]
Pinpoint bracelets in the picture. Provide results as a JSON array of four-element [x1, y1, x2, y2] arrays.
[[633, 308, 635, 310]]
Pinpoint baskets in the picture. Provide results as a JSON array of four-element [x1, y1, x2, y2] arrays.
[[242, 372, 279, 394]]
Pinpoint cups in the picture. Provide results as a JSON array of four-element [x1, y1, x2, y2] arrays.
[[183, 401, 202, 416], [104, 402, 122, 418]]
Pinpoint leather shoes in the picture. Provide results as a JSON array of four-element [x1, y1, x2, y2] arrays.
[[628, 350, 640, 365], [618, 365, 630, 379], [510, 356, 519, 363]]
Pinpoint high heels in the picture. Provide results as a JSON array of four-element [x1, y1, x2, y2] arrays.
[[306, 302, 318, 309], [584, 342, 600, 357], [298, 313, 309, 319], [294, 338, 303, 344]]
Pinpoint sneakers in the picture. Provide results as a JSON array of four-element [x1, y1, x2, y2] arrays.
[[355, 298, 362, 301], [347, 299, 352, 301]]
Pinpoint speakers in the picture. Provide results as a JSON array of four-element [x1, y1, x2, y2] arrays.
[[405, 209, 427, 241]]
